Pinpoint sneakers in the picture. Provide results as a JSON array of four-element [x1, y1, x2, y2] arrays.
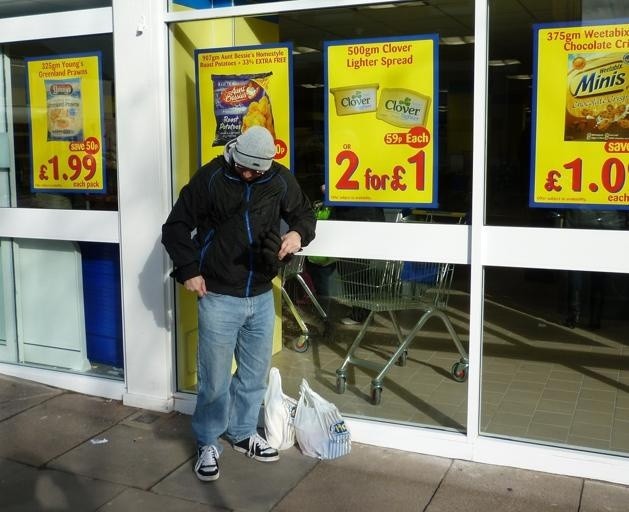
[[193, 445, 220, 481], [233, 433, 280, 462], [339, 317, 361, 324]]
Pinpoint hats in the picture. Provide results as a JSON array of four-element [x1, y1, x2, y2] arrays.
[[232, 127, 276, 171]]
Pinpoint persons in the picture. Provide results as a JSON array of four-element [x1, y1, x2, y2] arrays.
[[160, 126, 318, 481]]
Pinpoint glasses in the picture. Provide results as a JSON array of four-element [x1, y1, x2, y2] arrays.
[[235, 162, 265, 176]]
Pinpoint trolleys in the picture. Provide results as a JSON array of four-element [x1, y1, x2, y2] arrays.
[[278, 202, 337, 353], [336, 209, 469, 405]]
